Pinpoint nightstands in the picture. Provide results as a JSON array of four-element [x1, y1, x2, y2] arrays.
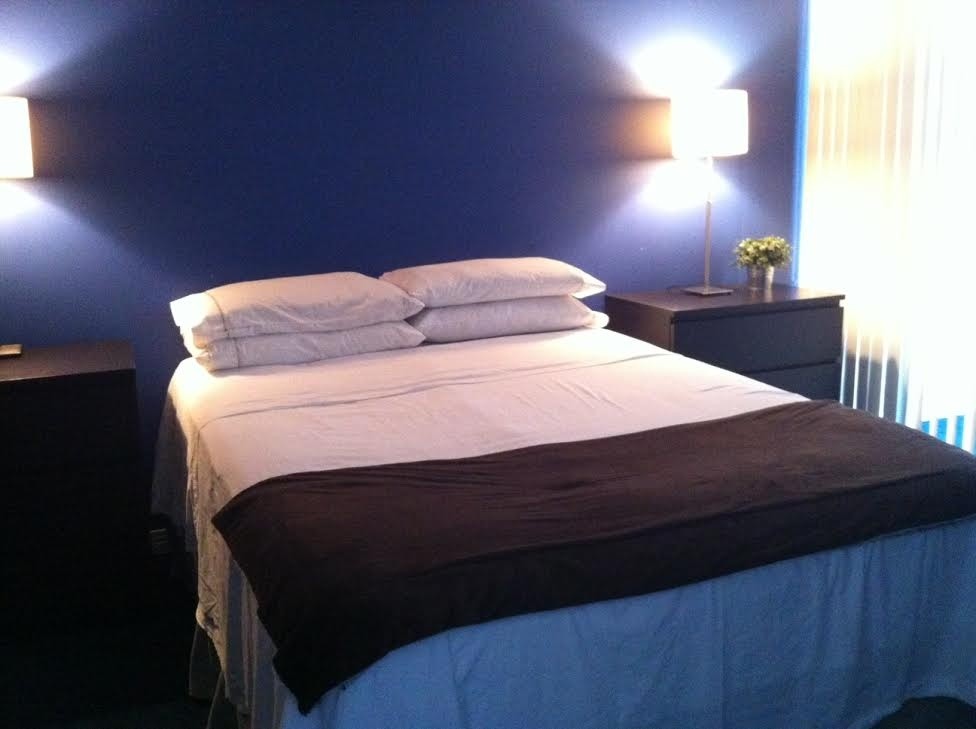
[[607, 281, 845, 404], [0, 341, 148, 544]]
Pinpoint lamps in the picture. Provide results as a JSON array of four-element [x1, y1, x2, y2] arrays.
[[0, 97, 34, 356], [672, 90, 748, 297]]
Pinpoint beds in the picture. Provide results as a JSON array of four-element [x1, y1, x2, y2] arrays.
[[151, 327, 976, 728]]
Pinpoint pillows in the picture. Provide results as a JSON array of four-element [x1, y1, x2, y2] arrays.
[[183, 324, 427, 370], [383, 254, 605, 307], [410, 294, 610, 343], [170, 274, 423, 341]]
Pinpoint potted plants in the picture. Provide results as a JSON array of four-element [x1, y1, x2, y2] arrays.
[[738, 237, 788, 293]]
